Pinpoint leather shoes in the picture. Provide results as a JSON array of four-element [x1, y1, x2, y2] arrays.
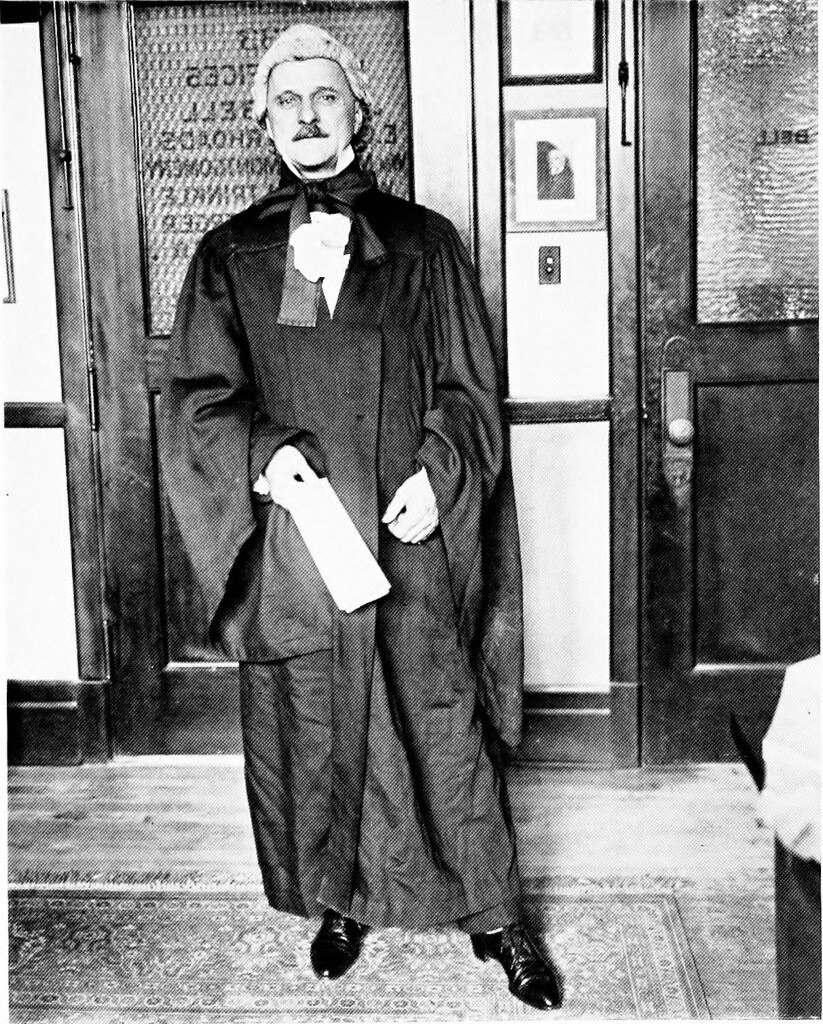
[[309, 907, 371, 978], [470, 922, 565, 1009]]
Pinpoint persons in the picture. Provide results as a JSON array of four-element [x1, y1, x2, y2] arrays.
[[537, 146, 574, 200], [155, 23, 563, 1010]]
